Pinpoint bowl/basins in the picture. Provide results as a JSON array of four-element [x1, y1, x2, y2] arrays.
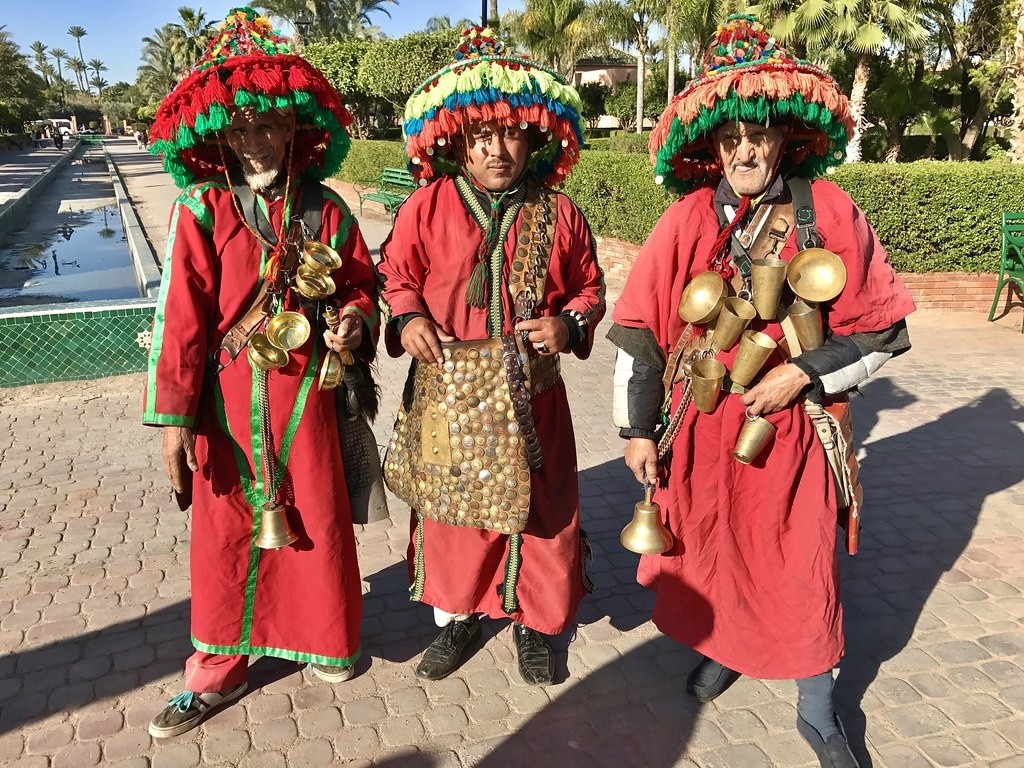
[[787, 247, 846, 304], [296, 240, 342, 301], [678, 271, 729, 325], [248, 311, 311, 373], [317, 350, 347, 390]]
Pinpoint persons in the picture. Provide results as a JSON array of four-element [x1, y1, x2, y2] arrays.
[[606, 13, 917, 768], [134, 129, 148, 149], [32, 130, 42, 148], [51, 126, 63, 145], [374, 20, 606, 686], [143, 7, 381, 740]]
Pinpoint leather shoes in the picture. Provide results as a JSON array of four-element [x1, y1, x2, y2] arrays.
[[511, 624, 555, 687], [417, 616, 481, 681]]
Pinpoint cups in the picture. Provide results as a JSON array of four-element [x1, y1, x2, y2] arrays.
[[786, 301, 825, 351], [733, 406, 776, 465], [730, 329, 778, 387], [750, 259, 788, 319], [691, 349, 727, 413], [713, 295, 757, 351]]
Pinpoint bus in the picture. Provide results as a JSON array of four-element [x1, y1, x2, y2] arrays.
[[24, 119, 73, 136]]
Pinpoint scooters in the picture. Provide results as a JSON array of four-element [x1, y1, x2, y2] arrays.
[[51, 135, 63, 150]]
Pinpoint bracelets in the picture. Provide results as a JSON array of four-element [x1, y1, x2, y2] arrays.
[[558, 309, 587, 346]]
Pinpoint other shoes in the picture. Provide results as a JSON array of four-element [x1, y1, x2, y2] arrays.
[[687, 654, 735, 703], [796, 709, 859, 768]]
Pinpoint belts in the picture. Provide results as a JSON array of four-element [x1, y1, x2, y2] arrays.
[[528, 349, 562, 399]]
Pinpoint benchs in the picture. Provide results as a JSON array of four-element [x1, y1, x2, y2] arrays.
[[987, 212, 1024, 335], [353, 168, 418, 226], [76, 135, 107, 145]]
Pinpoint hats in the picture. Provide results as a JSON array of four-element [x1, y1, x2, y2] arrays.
[[150, 7, 352, 190], [650, 13, 853, 194], [400, 25, 589, 190]]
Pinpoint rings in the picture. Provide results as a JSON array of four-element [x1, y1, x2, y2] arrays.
[[536, 342, 546, 352]]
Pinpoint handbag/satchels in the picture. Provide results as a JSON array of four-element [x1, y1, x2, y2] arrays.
[[379, 334, 530, 534], [826, 393, 864, 554]]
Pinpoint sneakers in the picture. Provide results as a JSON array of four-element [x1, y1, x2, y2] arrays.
[[314, 662, 355, 684], [148, 680, 248, 739]]
